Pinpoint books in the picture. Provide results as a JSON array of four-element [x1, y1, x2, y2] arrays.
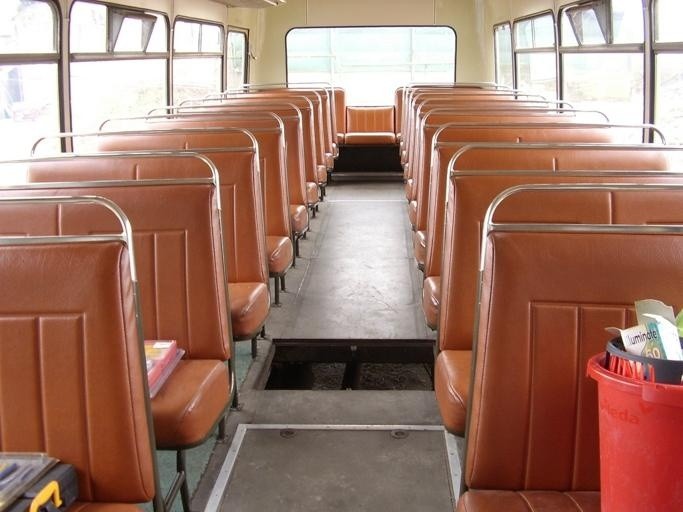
[[143, 337, 177, 387], [149, 347, 186, 398]]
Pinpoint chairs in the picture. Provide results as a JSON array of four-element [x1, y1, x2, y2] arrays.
[[395, 82, 683, 336], [0, 150, 244, 512], [433, 142, 683, 442], [458, 181, 683, 511], [27, 83, 345, 360], [344, 105, 396, 145]]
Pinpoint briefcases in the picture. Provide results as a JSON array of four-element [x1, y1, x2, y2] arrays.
[[6, 464, 81, 512]]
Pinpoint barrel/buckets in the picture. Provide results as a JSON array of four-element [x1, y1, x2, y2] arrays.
[[585, 353, 683, 512]]
